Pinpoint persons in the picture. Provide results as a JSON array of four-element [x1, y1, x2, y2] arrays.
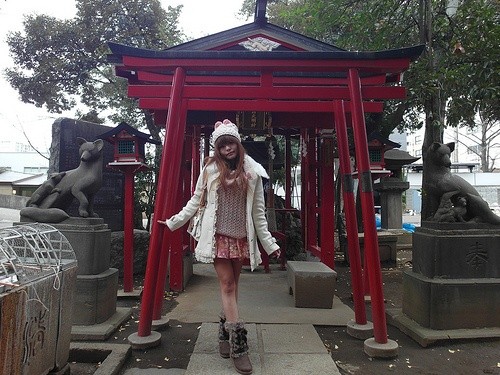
[[157, 119, 281, 374]]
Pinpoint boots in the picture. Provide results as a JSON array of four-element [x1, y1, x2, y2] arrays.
[[224, 319, 253, 375], [216, 311, 231, 358]]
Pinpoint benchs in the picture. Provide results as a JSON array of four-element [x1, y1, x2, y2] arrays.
[[287, 260, 337, 309]]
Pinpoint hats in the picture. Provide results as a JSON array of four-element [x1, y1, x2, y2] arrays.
[[210, 119, 241, 147]]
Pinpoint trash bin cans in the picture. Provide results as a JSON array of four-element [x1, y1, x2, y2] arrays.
[[0, 224, 78, 375]]
